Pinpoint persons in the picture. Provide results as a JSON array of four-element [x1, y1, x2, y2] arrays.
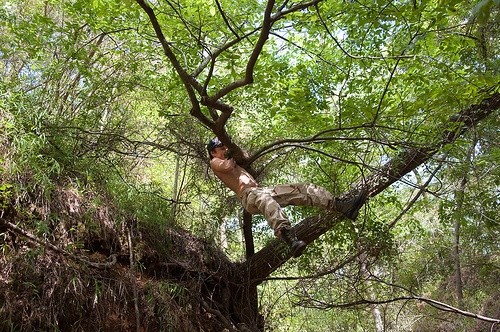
[[206, 136, 369, 258]]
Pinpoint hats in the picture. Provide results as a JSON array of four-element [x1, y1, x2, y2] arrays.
[[208, 140, 223, 151]]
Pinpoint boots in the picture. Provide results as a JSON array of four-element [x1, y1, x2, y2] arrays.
[[336, 192, 365, 220], [282, 227, 306, 258]]
